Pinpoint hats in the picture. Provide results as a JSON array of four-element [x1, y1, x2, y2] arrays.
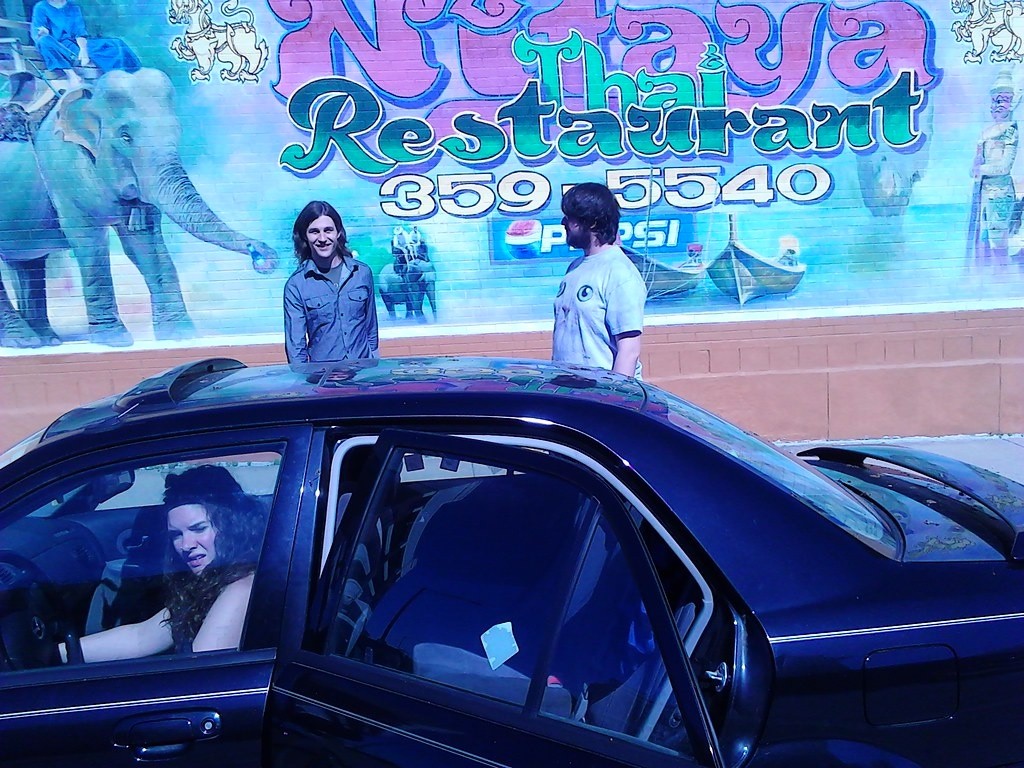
[[165, 464, 244, 513]]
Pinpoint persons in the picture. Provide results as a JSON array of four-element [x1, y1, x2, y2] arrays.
[[284, 201, 379, 363], [552, 183, 647, 380], [4, 465, 266, 666]]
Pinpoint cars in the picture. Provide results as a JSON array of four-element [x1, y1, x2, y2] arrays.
[[0, 355, 1024, 768]]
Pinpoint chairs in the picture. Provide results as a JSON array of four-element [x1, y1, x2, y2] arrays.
[[584, 505, 731, 746], [342, 445, 394, 552]]
